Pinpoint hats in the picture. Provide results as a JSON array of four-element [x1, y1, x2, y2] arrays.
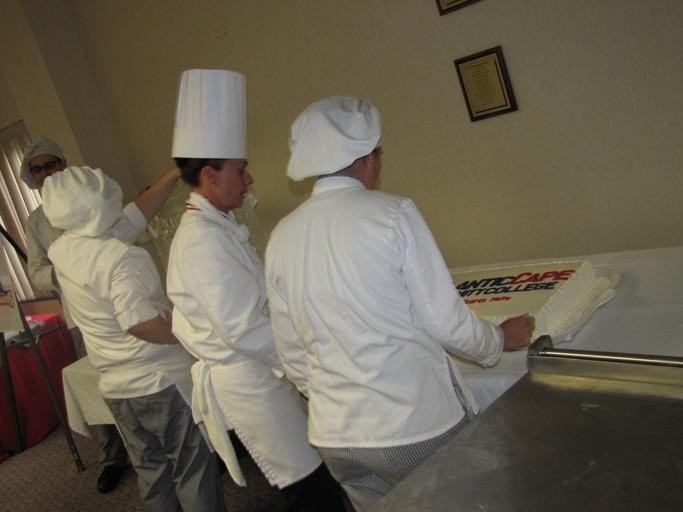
[[285, 95, 384, 182], [41, 165, 124, 237], [171, 68, 249, 160], [21, 135, 67, 190]]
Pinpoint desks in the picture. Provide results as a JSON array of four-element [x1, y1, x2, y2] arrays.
[[0, 311, 60, 464]]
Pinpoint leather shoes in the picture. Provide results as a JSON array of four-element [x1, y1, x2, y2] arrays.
[[97, 464, 122, 493]]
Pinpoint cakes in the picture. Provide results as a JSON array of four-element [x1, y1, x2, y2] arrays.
[[441, 257, 611, 346]]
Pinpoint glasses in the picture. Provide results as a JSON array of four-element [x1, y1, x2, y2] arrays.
[[31, 161, 57, 175]]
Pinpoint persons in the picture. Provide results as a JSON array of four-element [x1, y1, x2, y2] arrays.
[[39, 162, 231, 511], [261, 93, 537, 510], [19, 132, 128, 494], [164, 159, 349, 511]]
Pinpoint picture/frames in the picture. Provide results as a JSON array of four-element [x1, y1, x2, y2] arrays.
[[452, 43, 519, 123]]
